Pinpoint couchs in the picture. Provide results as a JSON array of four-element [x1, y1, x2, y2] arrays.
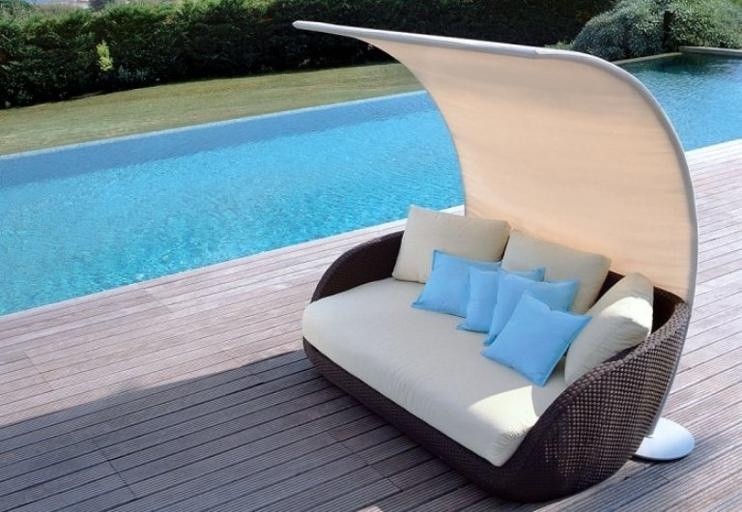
[[302, 230, 690, 504]]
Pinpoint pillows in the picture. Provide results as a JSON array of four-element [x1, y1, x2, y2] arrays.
[[410, 250, 593, 387]]
